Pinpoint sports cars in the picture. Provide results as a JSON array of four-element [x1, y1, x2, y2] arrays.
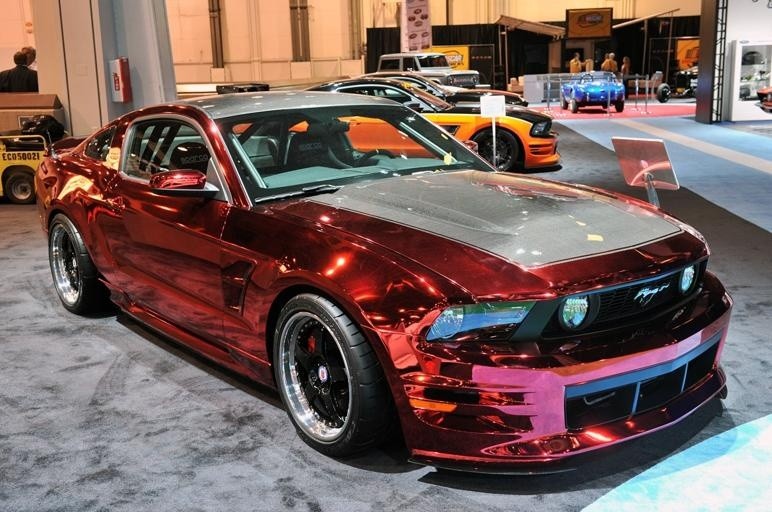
[[32, 90, 734, 476], [559, 71, 625, 114]]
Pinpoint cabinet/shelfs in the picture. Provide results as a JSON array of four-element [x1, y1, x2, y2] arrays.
[[726, 39, 772, 121]]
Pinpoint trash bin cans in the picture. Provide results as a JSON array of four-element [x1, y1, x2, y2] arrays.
[[215, 83, 269, 96]]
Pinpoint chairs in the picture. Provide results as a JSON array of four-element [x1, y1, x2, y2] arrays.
[[170, 140, 209, 176], [286, 121, 354, 168]]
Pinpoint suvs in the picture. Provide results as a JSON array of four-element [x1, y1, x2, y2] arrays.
[[656, 64, 700, 103], [377, 50, 481, 88]]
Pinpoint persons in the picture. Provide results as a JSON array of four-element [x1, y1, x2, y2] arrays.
[[22, 47, 39, 73], [1, 47, 38, 93], [569, 52, 632, 99]]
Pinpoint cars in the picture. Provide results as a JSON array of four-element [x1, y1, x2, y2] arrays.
[[754, 87, 771, 114]]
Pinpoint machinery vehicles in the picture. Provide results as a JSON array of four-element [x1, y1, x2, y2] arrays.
[[1, 129, 71, 204]]
